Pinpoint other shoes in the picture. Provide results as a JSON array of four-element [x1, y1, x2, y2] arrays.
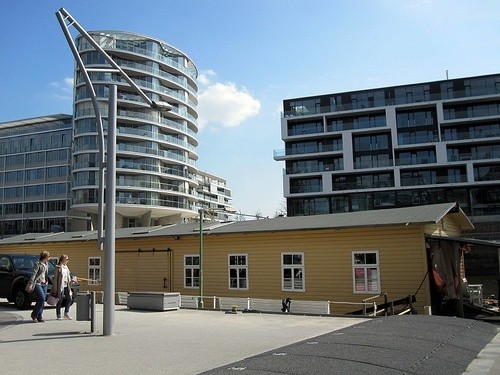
[[32, 316, 38, 322], [58, 317, 62, 319], [38, 319, 45, 322], [65, 313, 73, 319]]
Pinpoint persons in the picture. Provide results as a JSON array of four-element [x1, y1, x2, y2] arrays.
[[52, 254, 73, 320], [28, 251, 51, 323]]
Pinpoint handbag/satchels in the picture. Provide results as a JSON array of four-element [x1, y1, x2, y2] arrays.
[[26, 283, 35, 293], [47, 294, 60, 305]]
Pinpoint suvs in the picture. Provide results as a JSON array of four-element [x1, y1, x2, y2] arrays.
[[0, 254, 80, 311]]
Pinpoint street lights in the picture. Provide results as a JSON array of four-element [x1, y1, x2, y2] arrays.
[[197, 207, 237, 309], [56, 6, 173, 337]]
